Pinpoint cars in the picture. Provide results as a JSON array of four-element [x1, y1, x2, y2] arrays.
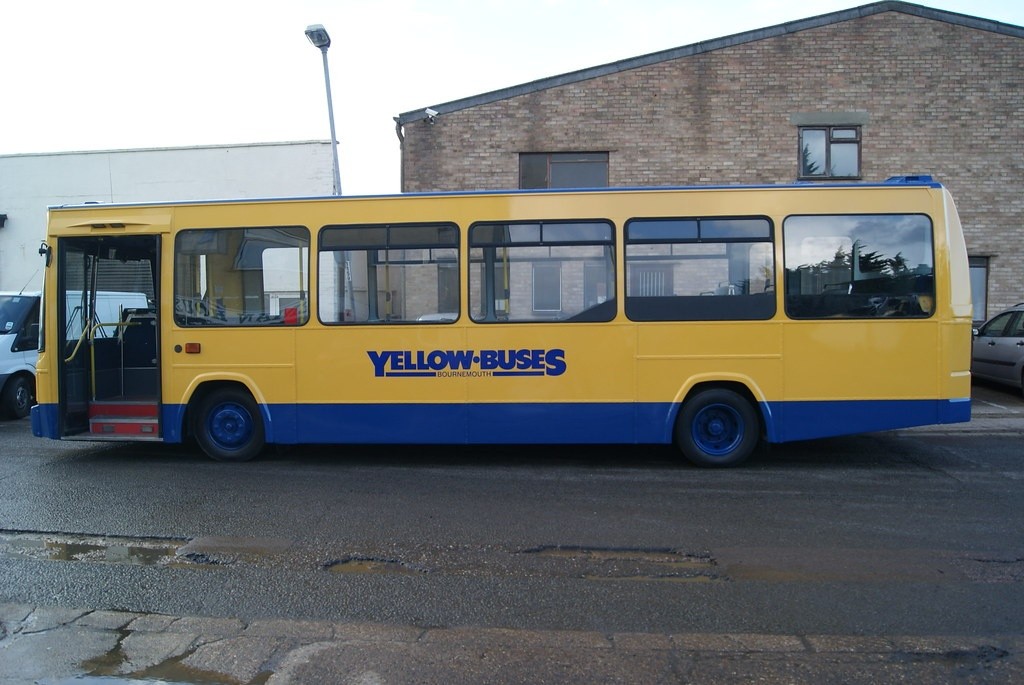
[[417, 312, 458, 324], [970, 303, 1023, 396]]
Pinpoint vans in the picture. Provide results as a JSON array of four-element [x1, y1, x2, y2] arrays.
[[0, 290, 149, 420]]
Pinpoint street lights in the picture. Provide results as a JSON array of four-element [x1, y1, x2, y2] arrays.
[[303, 24, 345, 323]]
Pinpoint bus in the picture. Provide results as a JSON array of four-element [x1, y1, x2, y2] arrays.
[[31, 173, 972, 468]]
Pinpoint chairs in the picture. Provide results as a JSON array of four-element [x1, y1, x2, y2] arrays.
[[822, 283, 854, 295], [714, 281, 736, 296]]
